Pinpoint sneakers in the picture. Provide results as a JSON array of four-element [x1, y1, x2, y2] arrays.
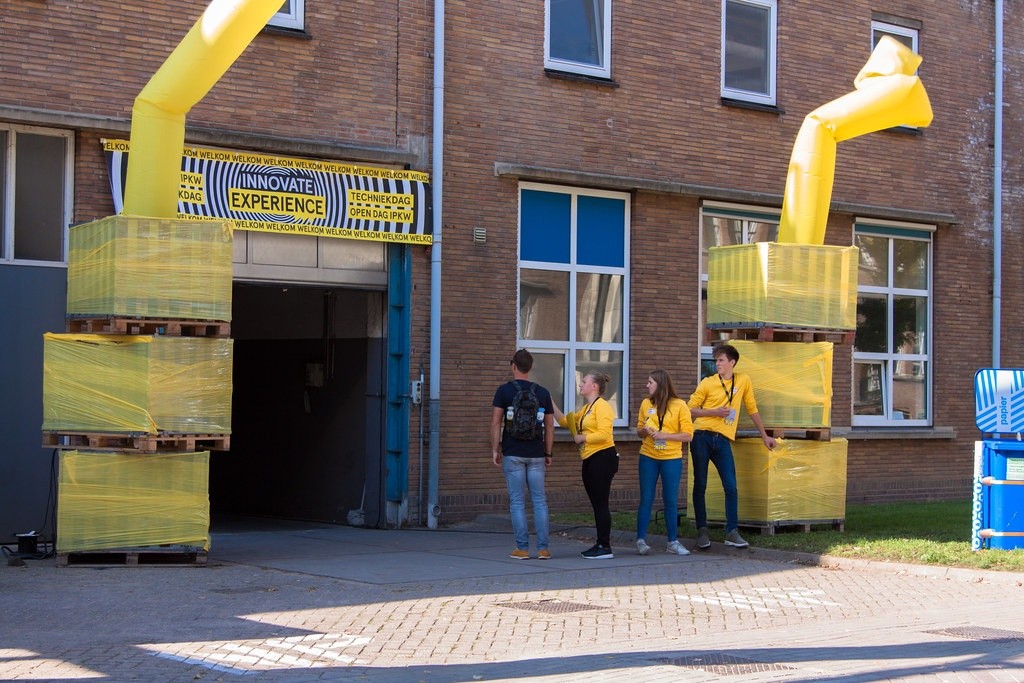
[[725, 528, 749, 548], [666, 540, 690, 556], [636, 539, 650, 554], [697, 527, 710, 547], [580, 544, 613, 559]]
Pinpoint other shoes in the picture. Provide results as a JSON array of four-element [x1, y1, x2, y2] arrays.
[[538, 550, 551, 559], [510, 549, 529, 560]]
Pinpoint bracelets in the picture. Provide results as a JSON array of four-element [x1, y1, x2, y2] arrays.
[[546, 452, 553, 458]]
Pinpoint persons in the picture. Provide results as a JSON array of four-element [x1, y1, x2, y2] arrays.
[[551, 370, 620, 559], [490, 349, 554, 560], [636, 370, 694, 555], [686, 345, 776, 548]]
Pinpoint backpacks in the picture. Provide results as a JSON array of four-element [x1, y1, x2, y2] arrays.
[[504, 380, 544, 440]]
